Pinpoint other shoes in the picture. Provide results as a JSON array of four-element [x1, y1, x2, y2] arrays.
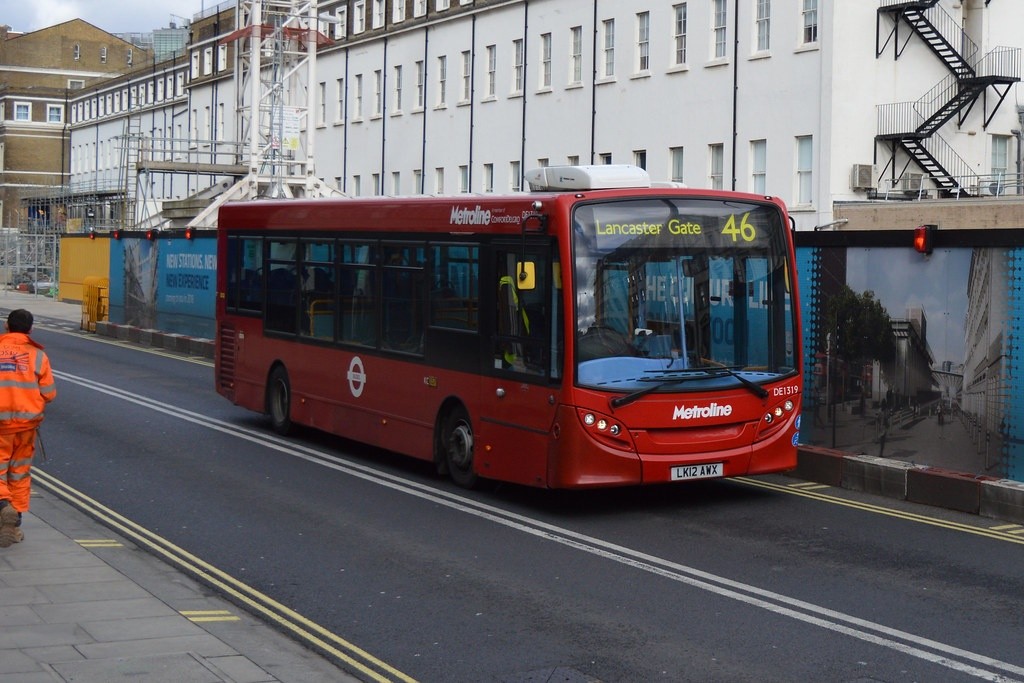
[[0, 500, 22, 548]]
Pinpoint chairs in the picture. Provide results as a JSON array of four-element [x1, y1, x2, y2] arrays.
[[232, 268, 458, 353]]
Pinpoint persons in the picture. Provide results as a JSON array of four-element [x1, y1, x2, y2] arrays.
[[0, 309, 57, 548], [814, 384, 947, 438]]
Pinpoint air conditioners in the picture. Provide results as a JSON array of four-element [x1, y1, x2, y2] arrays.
[[853, 164, 878, 189], [903, 173, 924, 190]]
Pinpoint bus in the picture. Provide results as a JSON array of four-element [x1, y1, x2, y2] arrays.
[[216, 165, 804, 494]]
